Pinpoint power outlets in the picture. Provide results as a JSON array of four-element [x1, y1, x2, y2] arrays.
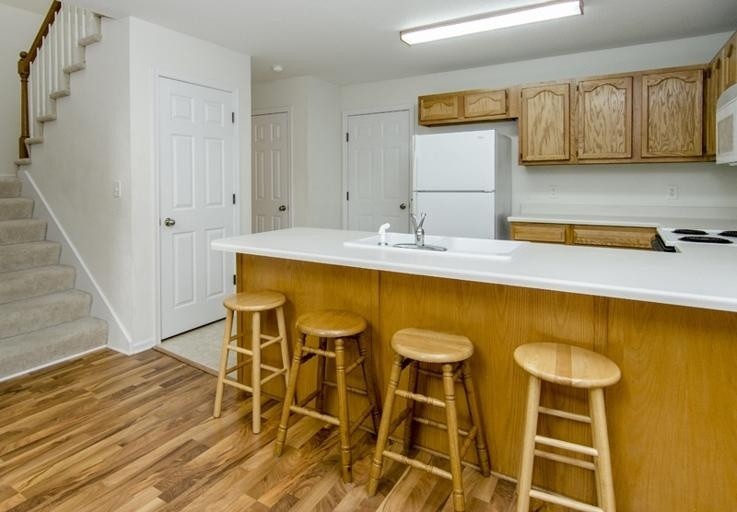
[[666, 185, 678, 202], [550, 184, 559, 200]]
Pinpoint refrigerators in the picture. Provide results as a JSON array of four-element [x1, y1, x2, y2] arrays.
[[407, 127, 513, 243]]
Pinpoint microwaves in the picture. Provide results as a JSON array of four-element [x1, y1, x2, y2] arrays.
[[713, 80, 737, 167]]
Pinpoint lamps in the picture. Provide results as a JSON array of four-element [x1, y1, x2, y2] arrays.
[[399, 1, 584, 47], [273, 64, 283, 73]]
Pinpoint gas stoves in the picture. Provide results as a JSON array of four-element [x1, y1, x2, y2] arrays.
[[654, 220, 737, 251]]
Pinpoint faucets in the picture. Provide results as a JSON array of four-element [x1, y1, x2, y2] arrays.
[[376, 222, 392, 245], [409, 210, 428, 246]]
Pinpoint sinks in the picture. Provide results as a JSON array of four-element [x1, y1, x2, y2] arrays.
[[344, 231, 462, 251]]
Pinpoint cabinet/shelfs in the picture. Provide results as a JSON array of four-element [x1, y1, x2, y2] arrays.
[[418, 89, 509, 126], [709, 31, 737, 164], [636, 64, 708, 162], [517, 71, 636, 165], [509, 221, 657, 250]]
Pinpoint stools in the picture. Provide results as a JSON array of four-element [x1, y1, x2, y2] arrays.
[[513, 342, 622, 512], [213, 289, 295, 434], [274, 309, 392, 483], [366, 327, 491, 512]]
